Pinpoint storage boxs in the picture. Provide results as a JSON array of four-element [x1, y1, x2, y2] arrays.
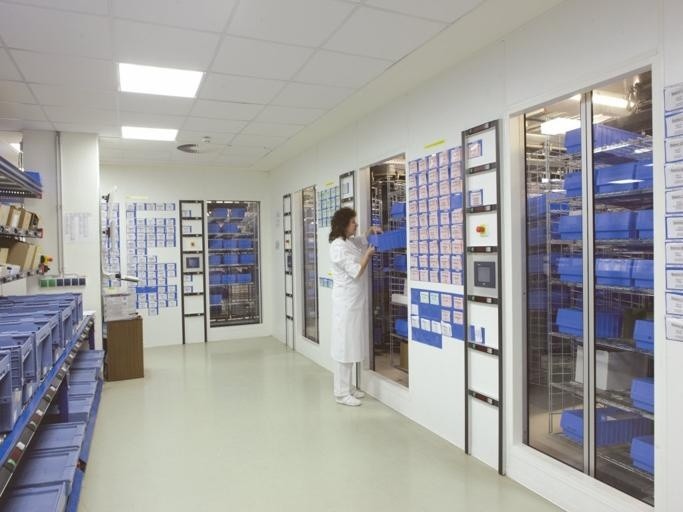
[[0, 202, 106, 512], [208, 207, 256, 307]]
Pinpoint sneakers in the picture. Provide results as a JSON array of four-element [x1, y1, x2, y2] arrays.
[[337, 396, 362, 406], [351, 391, 364, 398]]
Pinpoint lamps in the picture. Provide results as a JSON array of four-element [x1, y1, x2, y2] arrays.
[[540, 117, 582, 135], [574, 91, 636, 111], [102, 268, 139, 283]]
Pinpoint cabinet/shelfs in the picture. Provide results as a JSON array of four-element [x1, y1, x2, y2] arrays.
[[370, 178, 412, 372], [207, 201, 261, 327], [0, 155, 105, 512], [525, 108, 653, 493], [104, 308, 144, 380]]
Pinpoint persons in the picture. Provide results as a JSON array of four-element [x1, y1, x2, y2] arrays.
[[329, 208, 384, 407]]
